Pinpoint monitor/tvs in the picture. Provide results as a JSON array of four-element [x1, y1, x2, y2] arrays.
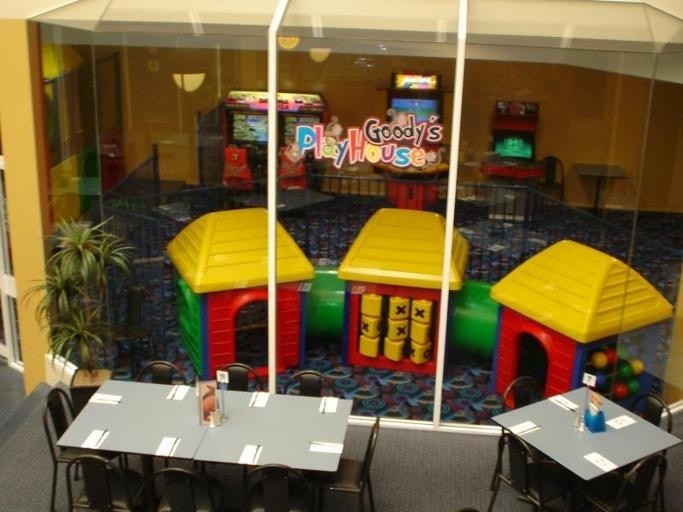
[[494, 129, 533, 162], [282, 117, 320, 153], [391, 92, 439, 131], [234, 112, 277, 152]]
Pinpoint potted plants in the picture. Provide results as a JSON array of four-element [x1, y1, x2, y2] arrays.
[[20, 215, 142, 416]]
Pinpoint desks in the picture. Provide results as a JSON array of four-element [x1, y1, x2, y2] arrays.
[[55, 379, 355, 512], [492, 384, 682, 511]]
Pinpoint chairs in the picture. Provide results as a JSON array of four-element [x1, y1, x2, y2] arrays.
[[323, 416, 381, 512], [138, 468, 217, 512], [488, 434, 578, 512], [577, 454, 668, 512], [135, 360, 184, 384], [215, 362, 263, 391], [64, 455, 131, 512], [283, 370, 338, 398], [626, 392, 674, 510], [491, 375, 543, 489], [244, 465, 317, 512], [42, 386, 76, 512]]
